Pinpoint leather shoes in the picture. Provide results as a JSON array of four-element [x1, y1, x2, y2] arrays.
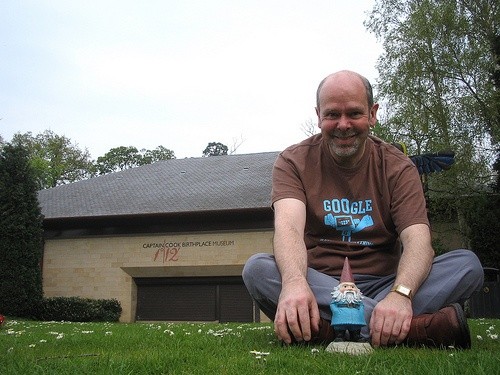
[[396, 302, 471, 350], [285, 316, 335, 347]]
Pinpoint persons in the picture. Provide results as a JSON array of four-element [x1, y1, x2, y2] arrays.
[[242, 71, 484, 351]]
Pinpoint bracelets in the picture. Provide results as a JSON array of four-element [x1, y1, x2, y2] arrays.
[[390, 283, 415, 302]]
